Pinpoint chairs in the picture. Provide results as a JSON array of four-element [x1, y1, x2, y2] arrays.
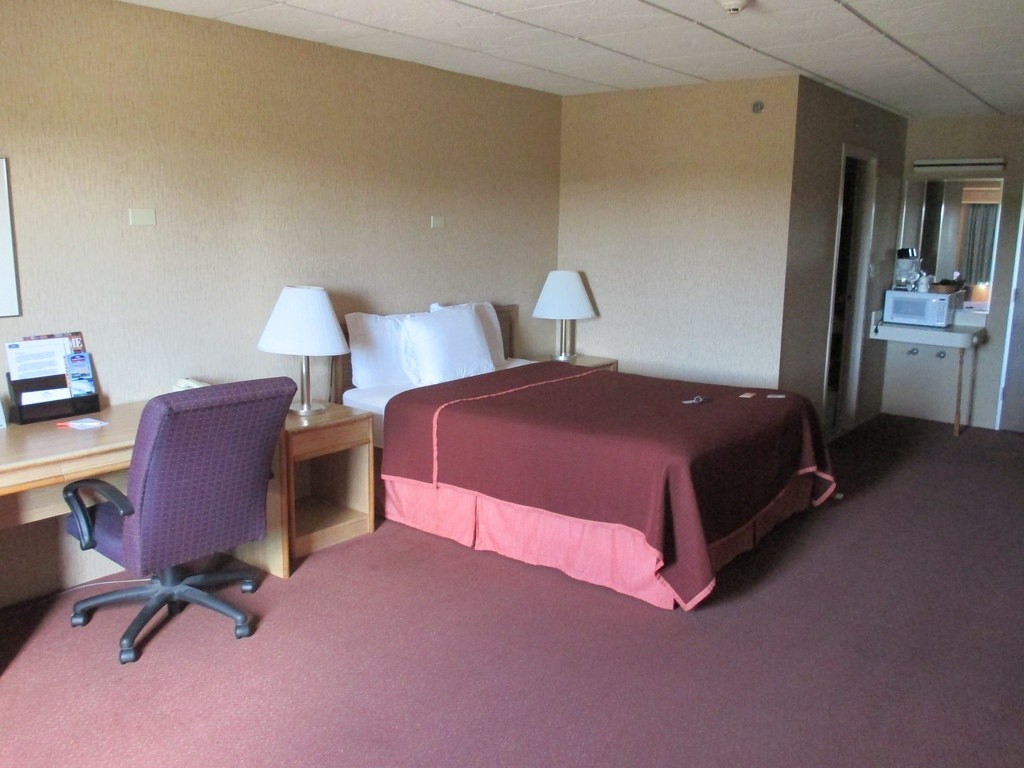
[[64, 375, 298, 667]]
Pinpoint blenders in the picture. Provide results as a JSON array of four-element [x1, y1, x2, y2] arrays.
[[892, 248, 920, 292]]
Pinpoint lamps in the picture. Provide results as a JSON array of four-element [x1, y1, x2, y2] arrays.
[[531, 270, 598, 362], [255, 286, 350, 417]]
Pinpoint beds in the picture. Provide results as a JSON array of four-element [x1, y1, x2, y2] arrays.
[[332, 304, 840, 612]]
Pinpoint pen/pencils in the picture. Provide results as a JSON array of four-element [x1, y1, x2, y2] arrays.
[[56, 422, 101, 425]]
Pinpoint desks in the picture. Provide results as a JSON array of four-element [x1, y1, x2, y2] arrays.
[[0, 402, 291, 581], [870, 323, 987, 438]]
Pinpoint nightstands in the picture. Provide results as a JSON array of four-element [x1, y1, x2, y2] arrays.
[[533, 354, 618, 373], [285, 399, 376, 563]]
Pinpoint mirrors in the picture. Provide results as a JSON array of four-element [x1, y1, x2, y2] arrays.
[[896, 177, 1004, 315]]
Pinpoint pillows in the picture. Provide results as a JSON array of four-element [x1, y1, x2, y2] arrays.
[[342, 312, 429, 387], [429, 301, 507, 367], [399, 304, 496, 385]]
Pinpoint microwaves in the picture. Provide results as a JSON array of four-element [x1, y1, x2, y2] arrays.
[[883, 289, 957, 327]]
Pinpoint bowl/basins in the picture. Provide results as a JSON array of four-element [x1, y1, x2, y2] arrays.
[[931, 282, 959, 293]]
